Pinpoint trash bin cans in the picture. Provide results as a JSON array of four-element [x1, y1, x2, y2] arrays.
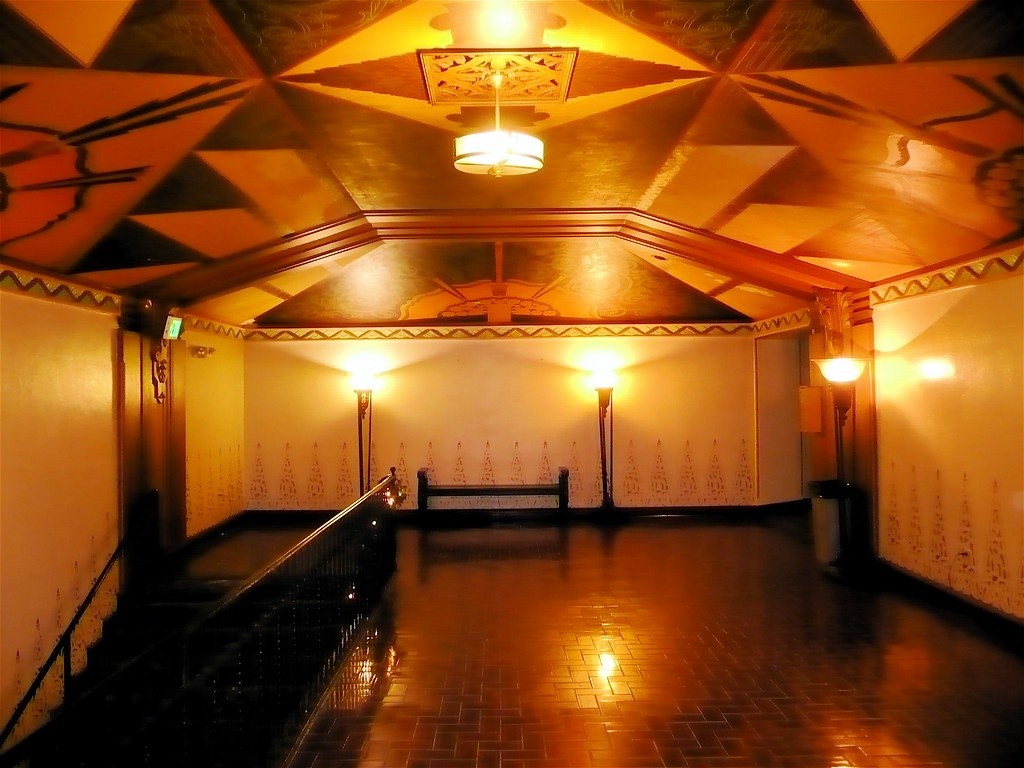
[[807, 480, 855, 565]]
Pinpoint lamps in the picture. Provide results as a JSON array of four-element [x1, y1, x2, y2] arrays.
[[808, 358, 869, 583], [194, 346, 215, 358], [451, 76, 544, 176]]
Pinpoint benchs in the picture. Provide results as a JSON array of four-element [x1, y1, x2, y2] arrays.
[[415, 468, 569, 520]]
